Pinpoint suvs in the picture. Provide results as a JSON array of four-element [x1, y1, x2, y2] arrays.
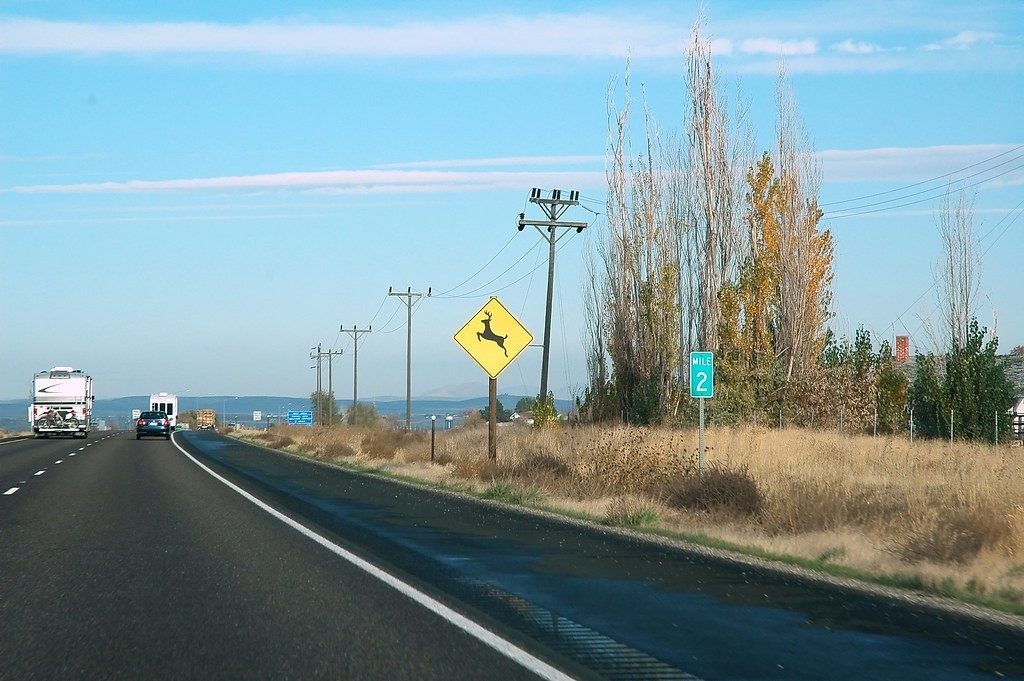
[[136, 411, 171, 441]]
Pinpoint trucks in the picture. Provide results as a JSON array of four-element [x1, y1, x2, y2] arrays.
[[149, 392, 179, 431]]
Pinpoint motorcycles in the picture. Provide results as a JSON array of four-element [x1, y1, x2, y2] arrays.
[[35, 408, 80, 430]]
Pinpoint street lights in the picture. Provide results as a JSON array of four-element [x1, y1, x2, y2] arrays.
[[175, 390, 190, 396], [267, 414, 271, 434], [196, 392, 212, 430], [230, 419, 231, 428], [223, 397, 239, 429], [235, 416, 238, 429]]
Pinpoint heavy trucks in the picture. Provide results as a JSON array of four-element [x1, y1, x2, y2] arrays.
[[27, 367, 94, 439]]
